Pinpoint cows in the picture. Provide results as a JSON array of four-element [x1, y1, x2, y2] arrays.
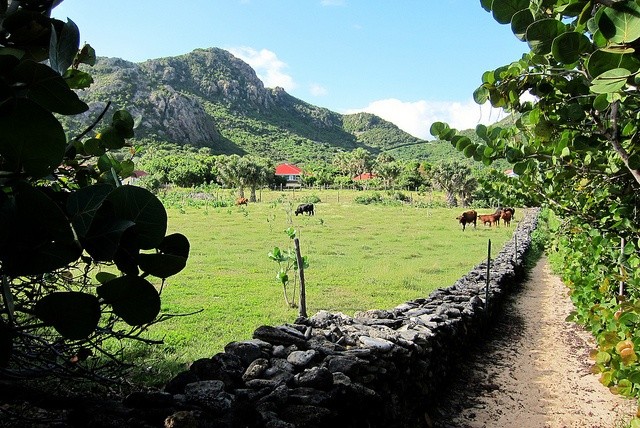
[[455, 209, 478, 232], [294, 203, 314, 216], [235, 197, 247, 206], [478, 206, 515, 226]]
